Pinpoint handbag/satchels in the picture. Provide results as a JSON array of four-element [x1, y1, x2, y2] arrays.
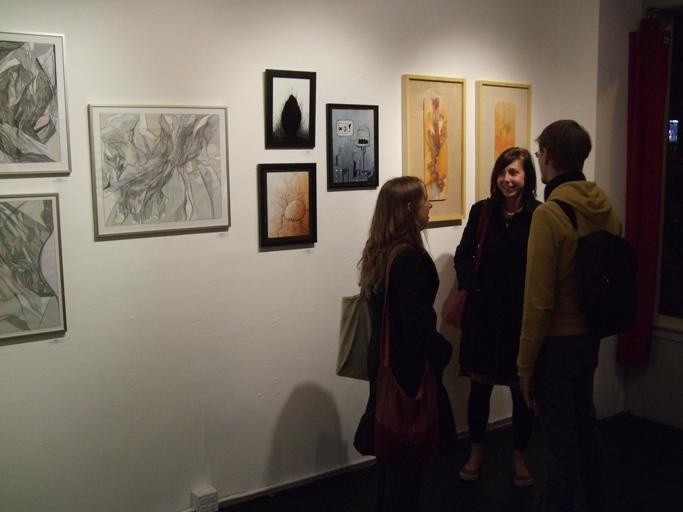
[[376, 368, 440, 472], [337, 294, 372, 381], [440, 274, 467, 327]]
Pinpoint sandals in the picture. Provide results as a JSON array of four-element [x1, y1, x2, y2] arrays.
[[512, 462, 533, 489], [457, 462, 483, 482]]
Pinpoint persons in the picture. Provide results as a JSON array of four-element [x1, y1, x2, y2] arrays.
[[451, 147, 549, 491], [513, 115, 625, 511], [350, 172, 458, 511]]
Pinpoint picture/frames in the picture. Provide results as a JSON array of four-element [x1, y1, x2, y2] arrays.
[[262, 67, 318, 153], [323, 101, 379, 192], [0, 27, 73, 178], [256, 163, 318, 249], [398, 72, 468, 226], [474, 78, 533, 206], [0, 192, 67, 346], [84, 101, 231, 244]]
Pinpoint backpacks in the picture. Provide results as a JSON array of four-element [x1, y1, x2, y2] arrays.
[[553, 198, 637, 337]]
